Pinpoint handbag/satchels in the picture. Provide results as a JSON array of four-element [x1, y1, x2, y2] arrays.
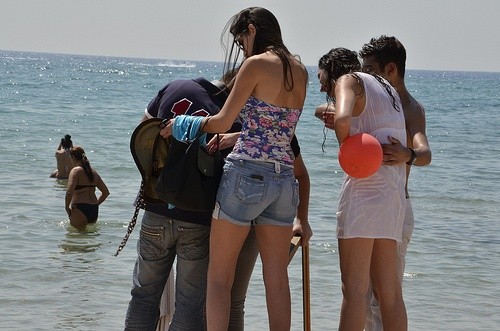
[[155, 119, 222, 213]]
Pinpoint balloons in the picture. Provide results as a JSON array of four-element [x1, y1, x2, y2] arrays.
[[338, 132, 384, 178]]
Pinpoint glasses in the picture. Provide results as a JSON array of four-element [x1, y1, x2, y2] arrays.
[[234, 28, 249, 50]]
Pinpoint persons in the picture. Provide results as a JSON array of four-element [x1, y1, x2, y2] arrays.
[[161, 7, 311, 331], [49, 35, 431, 331]]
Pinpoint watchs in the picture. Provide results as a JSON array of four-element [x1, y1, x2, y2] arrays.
[[406, 147, 417, 165]]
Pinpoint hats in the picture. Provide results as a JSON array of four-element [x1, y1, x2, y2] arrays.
[[130, 118, 171, 178]]
